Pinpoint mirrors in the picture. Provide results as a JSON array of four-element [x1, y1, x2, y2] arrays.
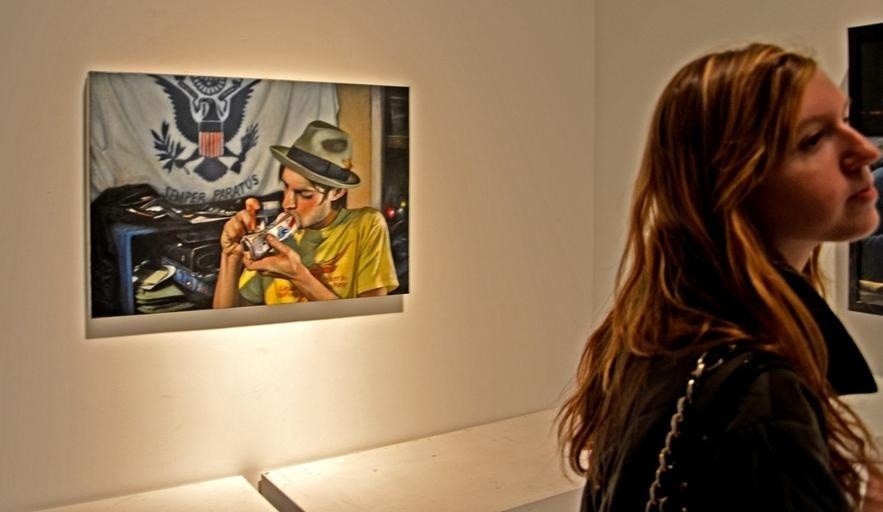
[[848, 22, 882, 316]]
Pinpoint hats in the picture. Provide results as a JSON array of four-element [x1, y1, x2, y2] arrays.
[[269, 120, 361, 189]]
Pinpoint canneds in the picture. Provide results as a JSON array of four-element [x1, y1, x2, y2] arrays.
[[241, 211, 301, 260]]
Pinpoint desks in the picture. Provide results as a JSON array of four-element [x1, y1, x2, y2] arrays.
[[260, 407, 593, 512], [39, 475, 279, 512]]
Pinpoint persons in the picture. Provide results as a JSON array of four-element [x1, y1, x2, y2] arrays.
[[548, 39, 882, 512], [210, 119, 401, 309]]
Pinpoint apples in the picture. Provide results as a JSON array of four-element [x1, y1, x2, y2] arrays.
[[384, 208, 396, 219]]
